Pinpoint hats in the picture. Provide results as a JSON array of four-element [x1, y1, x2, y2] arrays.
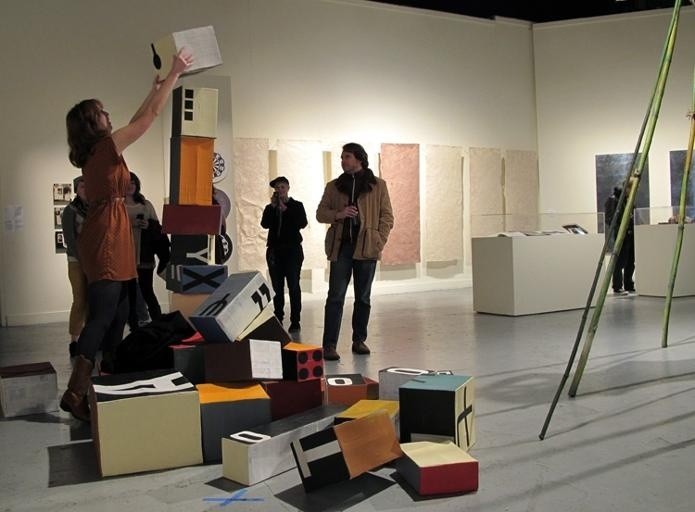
[[270, 176, 289, 186]]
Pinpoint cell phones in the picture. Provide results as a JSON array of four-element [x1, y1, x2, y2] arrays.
[[274, 192, 279, 206], [136, 214, 144, 228]]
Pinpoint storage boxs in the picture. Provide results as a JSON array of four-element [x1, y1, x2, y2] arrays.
[[189, 271, 275, 340], [109, 309, 197, 367], [263, 378, 325, 421], [398, 438, 479, 496], [379, 366, 431, 400], [148, 26, 223, 81], [88, 367, 203, 477], [399, 373, 476, 452], [162, 203, 222, 237], [171, 85, 219, 140], [171, 233, 216, 265], [223, 403, 349, 486], [206, 338, 283, 380], [194, 377, 270, 466], [0, 362, 59, 418], [290, 409, 404, 492], [165, 265, 226, 295], [283, 341, 324, 380], [326, 372, 378, 407], [170, 137, 215, 206]]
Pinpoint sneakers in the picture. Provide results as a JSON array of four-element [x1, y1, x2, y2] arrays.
[[289, 321, 299, 334], [352, 343, 369, 354], [324, 346, 340, 359]]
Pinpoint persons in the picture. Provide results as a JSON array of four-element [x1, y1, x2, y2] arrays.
[[59, 45, 196, 423], [62, 176, 88, 358], [212, 185, 227, 235], [316, 143, 394, 360], [260, 177, 308, 331], [606, 177, 640, 295], [126, 171, 162, 334]]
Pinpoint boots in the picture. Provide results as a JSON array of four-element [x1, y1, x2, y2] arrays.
[[59, 356, 93, 420]]
